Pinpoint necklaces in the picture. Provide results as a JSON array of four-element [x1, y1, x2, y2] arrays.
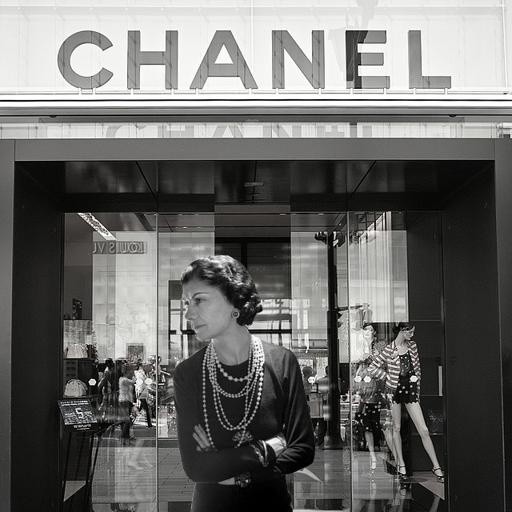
[[202, 334, 265, 451]]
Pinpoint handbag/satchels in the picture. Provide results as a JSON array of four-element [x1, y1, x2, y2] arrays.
[[65, 342, 90, 358], [63, 375, 89, 396]]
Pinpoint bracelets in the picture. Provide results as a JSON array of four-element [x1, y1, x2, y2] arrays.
[[234, 472, 249, 488], [275, 435, 287, 451]]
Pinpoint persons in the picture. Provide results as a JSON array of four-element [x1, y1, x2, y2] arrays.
[[314, 366, 345, 446], [300, 365, 318, 441], [100, 356, 172, 439], [173, 254, 315, 512], [362, 470, 441, 512], [356, 342, 399, 472], [368, 318, 446, 484]]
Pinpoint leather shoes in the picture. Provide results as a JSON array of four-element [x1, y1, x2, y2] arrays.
[[430, 467, 445, 484], [397, 462, 411, 482]]
